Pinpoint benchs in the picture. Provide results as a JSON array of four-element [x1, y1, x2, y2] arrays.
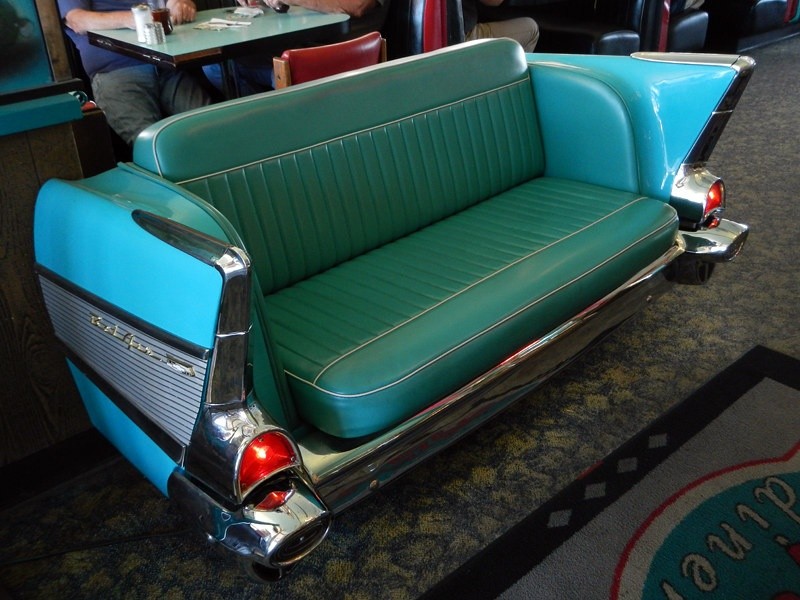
[[380, 0, 800, 61], [35, 41, 757, 584]]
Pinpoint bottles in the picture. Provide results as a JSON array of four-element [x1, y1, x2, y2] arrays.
[[131, 3, 154, 42], [144, 23, 166, 45], [151, 8, 173, 35]]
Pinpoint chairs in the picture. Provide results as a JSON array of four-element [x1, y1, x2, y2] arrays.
[[272, 31, 387, 91]]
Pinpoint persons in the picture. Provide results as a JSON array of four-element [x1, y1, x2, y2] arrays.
[[235, 0, 390, 100], [452, 0, 538, 53], [671, 0, 734, 53], [56, 0, 232, 160]]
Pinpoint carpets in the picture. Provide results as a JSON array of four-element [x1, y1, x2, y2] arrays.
[[416, 344, 800, 600]]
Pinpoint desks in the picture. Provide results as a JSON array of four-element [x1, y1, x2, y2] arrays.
[[88, 7, 350, 101]]
